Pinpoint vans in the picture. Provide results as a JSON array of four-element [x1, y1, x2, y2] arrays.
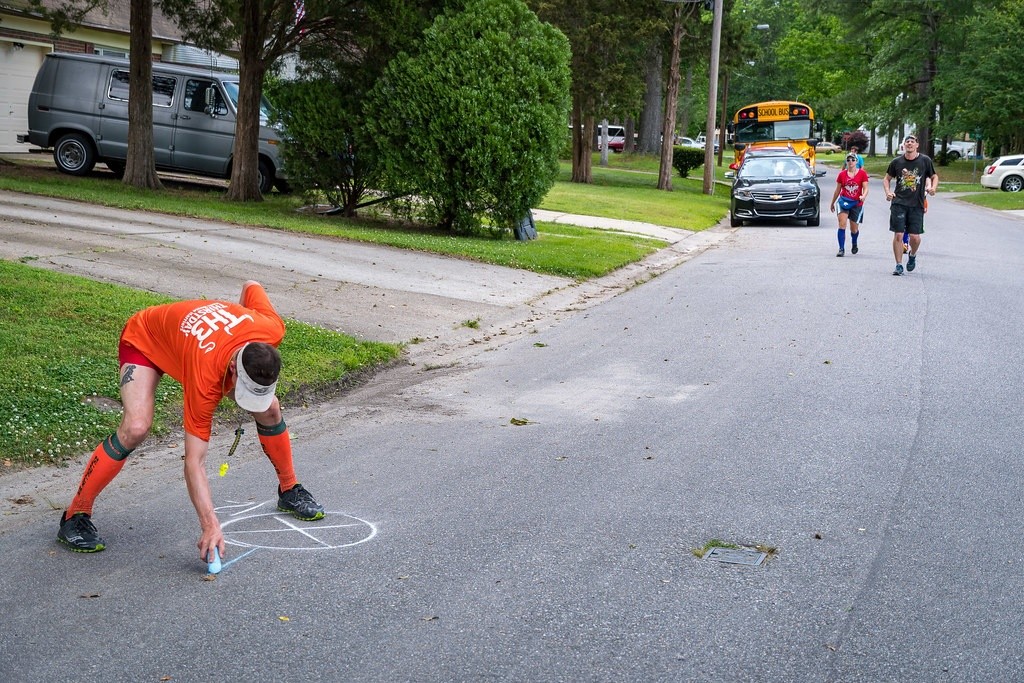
[[567, 125, 626, 153], [15, 53, 299, 195]]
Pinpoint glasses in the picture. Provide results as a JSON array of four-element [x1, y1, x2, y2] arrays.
[[847, 159, 856, 162]]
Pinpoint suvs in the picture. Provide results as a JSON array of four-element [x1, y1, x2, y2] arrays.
[[897, 135, 963, 161]]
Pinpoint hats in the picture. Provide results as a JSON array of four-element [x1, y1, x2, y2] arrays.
[[846, 152, 857, 160], [234, 342, 279, 413]]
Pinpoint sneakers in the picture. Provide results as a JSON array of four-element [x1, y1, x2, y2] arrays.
[[57, 510, 106, 552], [907, 250, 916, 271], [278, 482, 326, 520], [894, 264, 903, 275]]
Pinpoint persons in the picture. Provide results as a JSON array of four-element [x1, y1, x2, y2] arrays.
[[674, 133, 682, 146], [57, 277, 325, 563], [883, 135, 938, 276], [902, 178, 932, 254], [830, 154, 869, 257], [842, 146, 864, 171]]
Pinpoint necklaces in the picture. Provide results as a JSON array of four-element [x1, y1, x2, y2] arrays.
[[218, 349, 245, 477]]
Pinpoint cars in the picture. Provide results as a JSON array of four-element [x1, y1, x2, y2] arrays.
[[728, 143, 799, 170], [980, 154, 1024, 193], [698, 136, 719, 154], [815, 142, 842, 154], [677, 136, 705, 152], [724, 154, 823, 227]]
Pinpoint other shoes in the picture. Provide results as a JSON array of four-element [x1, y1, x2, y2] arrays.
[[852, 239, 858, 254], [837, 248, 844, 257], [903, 243, 908, 253]]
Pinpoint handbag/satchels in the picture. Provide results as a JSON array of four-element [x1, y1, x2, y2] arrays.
[[838, 197, 859, 209]]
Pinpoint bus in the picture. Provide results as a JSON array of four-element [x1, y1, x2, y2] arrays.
[[727, 101, 823, 178]]
